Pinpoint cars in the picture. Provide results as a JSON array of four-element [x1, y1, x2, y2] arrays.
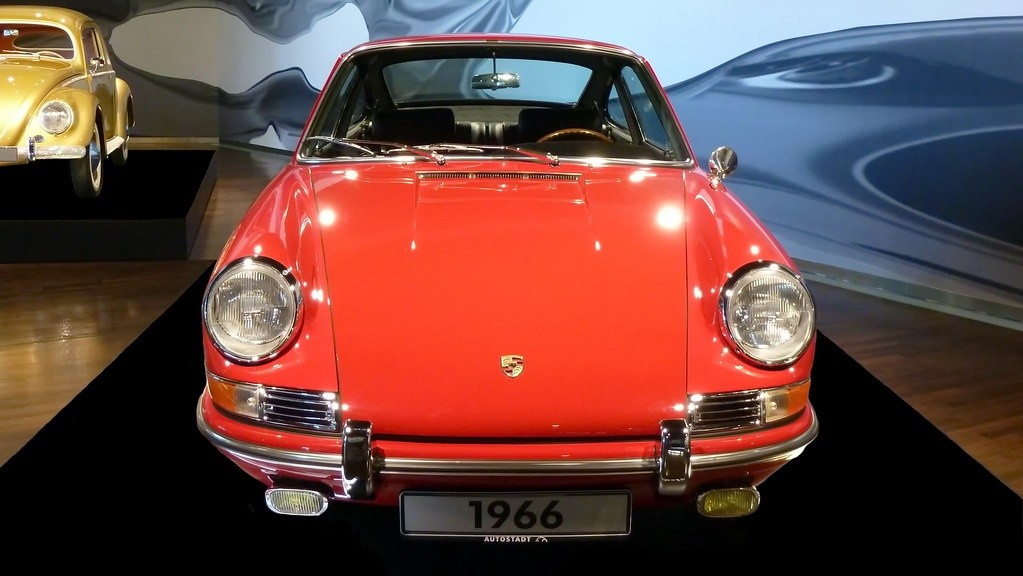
[[196, 33, 820, 535], [0, 5, 135, 197]]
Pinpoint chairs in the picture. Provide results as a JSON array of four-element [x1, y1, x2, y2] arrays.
[[519, 108, 603, 146], [372, 108, 457, 147]]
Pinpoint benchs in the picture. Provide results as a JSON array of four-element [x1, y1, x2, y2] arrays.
[[457, 122, 518, 145]]
